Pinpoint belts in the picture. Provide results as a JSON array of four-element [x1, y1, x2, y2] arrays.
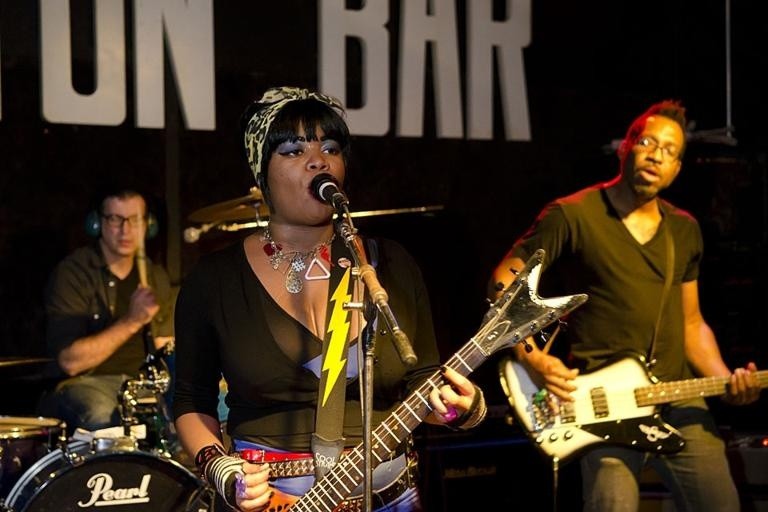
[[270, 457, 421, 512], [232, 448, 355, 463], [266, 434, 412, 480]]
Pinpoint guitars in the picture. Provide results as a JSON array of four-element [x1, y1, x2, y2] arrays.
[[499, 350, 768, 463], [288, 248, 588, 511]]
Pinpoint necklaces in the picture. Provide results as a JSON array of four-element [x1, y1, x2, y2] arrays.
[[258, 225, 339, 295]]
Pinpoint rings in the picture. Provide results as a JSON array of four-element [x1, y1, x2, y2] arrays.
[[434, 404, 458, 423], [234, 474, 248, 498]]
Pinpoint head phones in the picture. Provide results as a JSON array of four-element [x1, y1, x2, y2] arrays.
[[84, 165, 162, 240]]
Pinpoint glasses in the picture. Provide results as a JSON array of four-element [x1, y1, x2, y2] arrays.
[[100, 212, 146, 227], [631, 137, 683, 160]]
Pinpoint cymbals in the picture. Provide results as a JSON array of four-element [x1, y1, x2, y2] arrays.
[[0, 357, 50, 365], [187, 196, 271, 224]]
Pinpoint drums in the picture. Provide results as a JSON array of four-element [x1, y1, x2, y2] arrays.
[[0, 417, 66, 508], [4, 440, 221, 512]]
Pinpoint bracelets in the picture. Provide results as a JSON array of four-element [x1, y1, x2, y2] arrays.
[[194, 443, 229, 474]]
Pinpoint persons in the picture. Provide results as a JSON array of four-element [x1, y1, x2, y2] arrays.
[[487, 101, 759, 512], [174, 85, 507, 511], [37, 186, 180, 433]]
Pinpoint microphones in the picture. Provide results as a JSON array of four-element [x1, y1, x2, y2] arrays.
[[308, 173, 350, 235]]
